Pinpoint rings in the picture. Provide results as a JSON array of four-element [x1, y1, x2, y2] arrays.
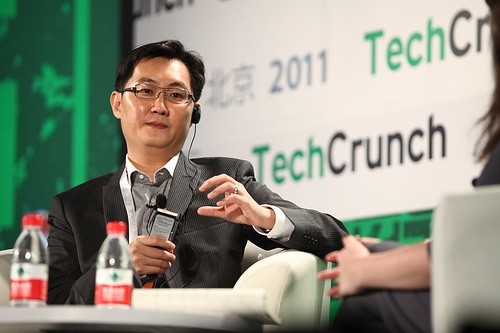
[[230, 183, 241, 195]]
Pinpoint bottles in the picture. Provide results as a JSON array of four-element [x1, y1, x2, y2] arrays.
[[10, 216, 48, 307], [95, 222, 134, 309]]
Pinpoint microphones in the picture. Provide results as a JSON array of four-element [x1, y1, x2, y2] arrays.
[[156, 194, 167, 209]]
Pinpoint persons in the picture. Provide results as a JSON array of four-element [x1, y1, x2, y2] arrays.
[[46, 39, 350, 307], [317, 1, 500, 333]]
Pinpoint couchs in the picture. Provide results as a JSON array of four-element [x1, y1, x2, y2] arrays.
[[0, 237, 333, 333], [432, 186, 499, 333]]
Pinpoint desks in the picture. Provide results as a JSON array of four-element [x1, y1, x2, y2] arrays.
[[1, 305, 263, 333]]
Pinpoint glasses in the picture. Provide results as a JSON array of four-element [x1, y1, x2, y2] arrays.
[[120, 84, 195, 104]]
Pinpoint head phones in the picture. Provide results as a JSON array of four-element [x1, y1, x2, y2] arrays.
[[191, 106, 200, 124]]
